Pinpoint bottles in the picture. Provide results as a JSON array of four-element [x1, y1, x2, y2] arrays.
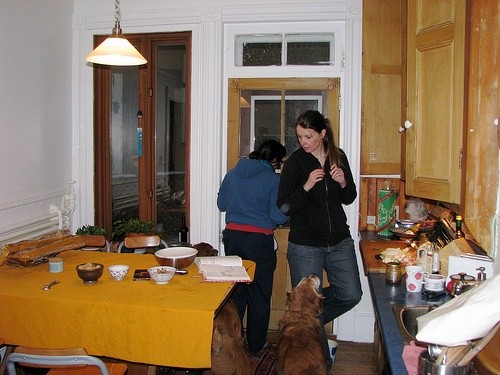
[[385, 262, 402, 283], [48, 258, 64, 273], [447, 273, 476, 297], [178, 214, 190, 244], [452, 215, 465, 242], [416, 233, 441, 274]]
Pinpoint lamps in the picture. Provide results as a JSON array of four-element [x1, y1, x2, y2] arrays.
[[83, 1, 148, 67]]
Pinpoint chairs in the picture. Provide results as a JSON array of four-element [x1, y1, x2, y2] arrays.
[[117, 235, 170, 254], [0, 344, 128, 375]]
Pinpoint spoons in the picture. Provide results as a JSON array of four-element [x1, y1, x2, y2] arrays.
[[41, 280, 60, 290]]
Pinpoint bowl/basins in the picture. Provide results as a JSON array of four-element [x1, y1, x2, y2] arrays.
[[75, 263, 104, 286], [108, 266, 129, 281], [155, 247, 198, 270], [148, 266, 176, 284]]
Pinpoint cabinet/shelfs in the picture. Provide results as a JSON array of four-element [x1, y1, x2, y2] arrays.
[[360, 0, 500, 259]]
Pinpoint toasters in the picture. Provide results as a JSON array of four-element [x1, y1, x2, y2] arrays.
[[447, 251, 495, 291]]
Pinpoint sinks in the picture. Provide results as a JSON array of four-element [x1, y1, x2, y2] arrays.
[[392, 303, 441, 346]]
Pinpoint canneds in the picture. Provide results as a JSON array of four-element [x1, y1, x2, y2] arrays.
[[385, 262, 402, 284]]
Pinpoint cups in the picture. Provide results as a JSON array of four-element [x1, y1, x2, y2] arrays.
[[404, 266, 429, 292], [418, 350, 478, 375], [424, 274, 445, 292]]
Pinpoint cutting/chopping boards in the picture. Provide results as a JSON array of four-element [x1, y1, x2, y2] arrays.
[[359, 237, 417, 273]]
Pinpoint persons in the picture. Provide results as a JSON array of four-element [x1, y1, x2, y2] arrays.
[[217, 140, 290, 352], [276, 110, 363, 371]]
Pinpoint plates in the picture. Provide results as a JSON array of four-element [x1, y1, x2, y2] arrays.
[[388, 228, 418, 239]]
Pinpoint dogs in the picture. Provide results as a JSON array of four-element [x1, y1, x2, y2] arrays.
[[191, 243, 253, 375], [276, 273, 331, 374]]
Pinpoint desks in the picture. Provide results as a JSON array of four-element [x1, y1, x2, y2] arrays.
[[0, 251, 256, 375]]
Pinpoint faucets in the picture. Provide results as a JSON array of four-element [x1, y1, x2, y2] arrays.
[[451, 267, 486, 296]]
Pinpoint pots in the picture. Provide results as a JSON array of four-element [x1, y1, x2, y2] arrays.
[[395, 219, 416, 228]]
